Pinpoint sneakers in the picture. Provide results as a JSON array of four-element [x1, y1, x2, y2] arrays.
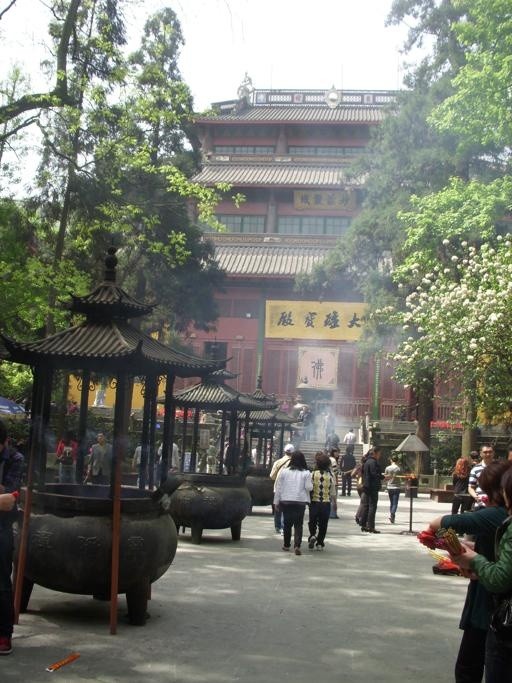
[[317, 544, 323, 550], [282, 544, 289, 550], [0, 635, 13, 653], [308, 535, 316, 548], [294, 546, 301, 554]]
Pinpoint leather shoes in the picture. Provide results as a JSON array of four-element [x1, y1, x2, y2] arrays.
[[275, 526, 280, 533]]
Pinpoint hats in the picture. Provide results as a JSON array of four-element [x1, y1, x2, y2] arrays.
[[284, 443, 295, 453]]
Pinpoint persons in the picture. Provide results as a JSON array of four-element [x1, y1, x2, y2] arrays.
[[355, 448, 401, 533], [270, 444, 340, 556], [429, 440, 512, 682], [69, 401, 79, 413], [132, 440, 179, 490], [339, 446, 356, 496], [1, 437, 23, 655], [281, 402, 289, 414], [296, 406, 334, 441], [57, 430, 112, 485], [325, 429, 356, 453], [197, 437, 230, 474]]
